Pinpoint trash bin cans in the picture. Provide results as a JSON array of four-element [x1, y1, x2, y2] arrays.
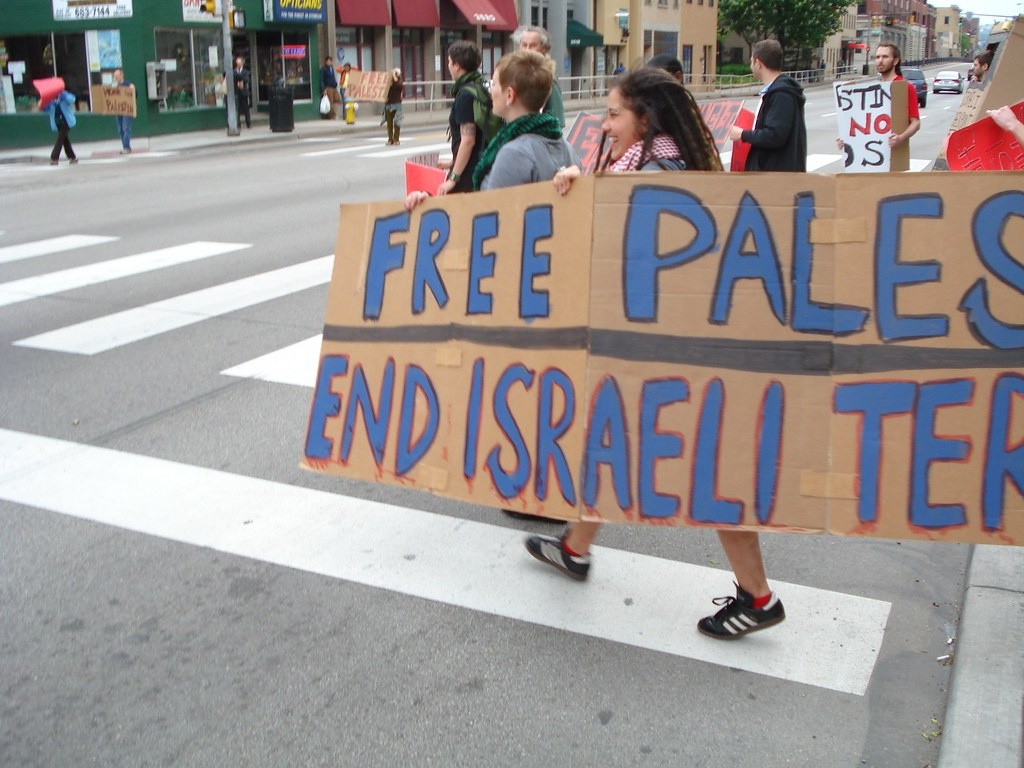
[[268, 85, 294, 133]]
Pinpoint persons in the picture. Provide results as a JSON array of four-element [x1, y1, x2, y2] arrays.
[[731, 38, 806, 173], [971, 51, 996, 82], [406, 25, 571, 527], [113, 69, 136, 155], [337, 64, 357, 120], [986, 106, 1024, 146], [320, 57, 337, 119], [523, 52, 786, 641], [837, 43, 921, 149], [222, 57, 251, 130], [384, 68, 407, 146], [40, 91, 78, 164]]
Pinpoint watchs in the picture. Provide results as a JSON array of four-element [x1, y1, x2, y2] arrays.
[[449, 172, 460, 183]]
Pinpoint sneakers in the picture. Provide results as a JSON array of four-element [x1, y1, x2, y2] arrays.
[[697, 582, 786, 640], [526, 537, 591, 581]]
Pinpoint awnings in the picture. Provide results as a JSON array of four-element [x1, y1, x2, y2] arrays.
[[336, 0, 518, 32], [567, 19, 604, 49]]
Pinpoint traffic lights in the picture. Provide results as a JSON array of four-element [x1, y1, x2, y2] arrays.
[[958, 18, 963, 26], [229, 9, 246, 30], [200, 0, 216, 16]]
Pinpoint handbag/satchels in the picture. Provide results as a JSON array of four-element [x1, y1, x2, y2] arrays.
[[320, 92, 330, 114]]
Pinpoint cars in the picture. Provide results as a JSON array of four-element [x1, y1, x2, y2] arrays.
[[968, 67, 974, 81], [900, 68, 928, 108], [932, 70, 965, 94]]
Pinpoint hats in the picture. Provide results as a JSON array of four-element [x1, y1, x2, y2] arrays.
[[393, 68, 401, 75]]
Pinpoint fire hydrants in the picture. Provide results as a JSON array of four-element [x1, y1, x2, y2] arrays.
[[344, 100, 360, 124]]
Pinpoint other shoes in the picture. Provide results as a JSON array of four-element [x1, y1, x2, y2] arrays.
[[387, 139, 400, 146], [50, 159, 58, 166], [70, 156, 78, 165], [120, 148, 130, 155]]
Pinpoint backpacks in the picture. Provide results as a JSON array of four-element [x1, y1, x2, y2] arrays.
[[463, 82, 505, 144]]
